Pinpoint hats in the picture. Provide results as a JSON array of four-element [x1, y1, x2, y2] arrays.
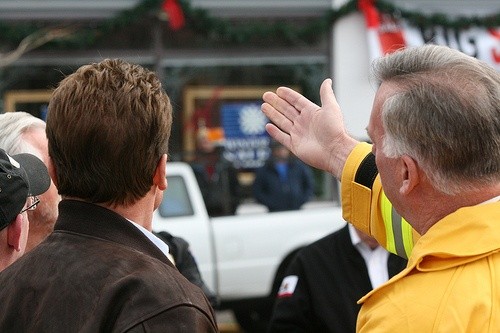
[[0, 149, 51, 231]]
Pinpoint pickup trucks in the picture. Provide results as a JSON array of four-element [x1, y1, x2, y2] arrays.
[[151, 162, 347, 333]]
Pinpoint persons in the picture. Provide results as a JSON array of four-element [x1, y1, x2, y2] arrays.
[[152, 231, 215, 308], [254, 141, 314, 213], [262, 44, 500, 333], [0, 58, 219, 333], [263, 139, 409, 333], [192, 128, 243, 218], [0, 112, 62, 273]]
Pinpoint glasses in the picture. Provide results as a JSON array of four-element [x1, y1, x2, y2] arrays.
[[20, 196, 40, 214]]
